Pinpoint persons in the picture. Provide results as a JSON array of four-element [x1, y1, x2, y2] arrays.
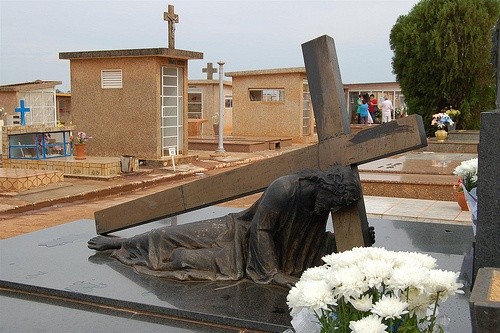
[[355, 93, 393, 124], [86, 165, 376, 290]]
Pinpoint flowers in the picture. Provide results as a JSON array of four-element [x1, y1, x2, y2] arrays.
[[212, 111, 219, 123], [70, 131, 89, 143], [288, 246, 461, 333], [452, 160, 483, 192], [391, 107, 400, 116], [431, 106, 460, 128], [376, 109, 383, 118]]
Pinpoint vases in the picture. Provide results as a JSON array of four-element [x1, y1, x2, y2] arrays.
[[74, 142, 88, 159], [457, 190, 469, 210], [213, 124, 220, 135], [435, 130, 449, 141], [392, 115, 397, 118], [464, 186, 480, 238], [375, 117, 381, 124], [446, 121, 457, 131]]
